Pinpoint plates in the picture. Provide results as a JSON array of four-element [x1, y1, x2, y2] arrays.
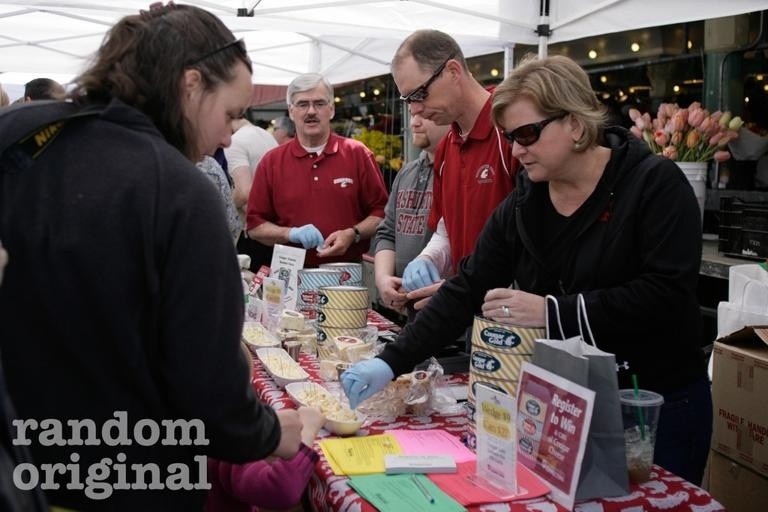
[[238, 321, 281, 352], [253, 347, 310, 387], [283, 381, 367, 436]]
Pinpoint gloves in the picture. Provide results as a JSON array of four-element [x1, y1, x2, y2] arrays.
[[340, 358, 394, 409], [289, 224, 324, 249], [401, 258, 441, 293]]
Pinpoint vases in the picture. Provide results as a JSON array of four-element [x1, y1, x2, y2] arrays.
[[674, 161, 708, 228]]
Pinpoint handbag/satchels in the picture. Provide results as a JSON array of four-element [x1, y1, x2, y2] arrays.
[[534, 293, 629, 500]]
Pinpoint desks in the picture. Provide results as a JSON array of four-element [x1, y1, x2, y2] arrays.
[[241, 274, 725, 512]]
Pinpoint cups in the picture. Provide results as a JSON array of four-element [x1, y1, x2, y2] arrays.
[[614, 388, 665, 482]]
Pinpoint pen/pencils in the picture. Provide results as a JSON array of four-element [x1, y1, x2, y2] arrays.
[[410, 472, 434, 503]]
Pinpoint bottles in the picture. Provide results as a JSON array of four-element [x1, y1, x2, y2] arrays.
[[239, 272, 249, 317]]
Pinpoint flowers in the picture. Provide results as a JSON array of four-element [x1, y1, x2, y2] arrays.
[[617, 96, 741, 169], [345, 124, 407, 174]]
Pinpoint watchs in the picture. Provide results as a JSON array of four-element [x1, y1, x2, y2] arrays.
[[352, 226, 360, 244]]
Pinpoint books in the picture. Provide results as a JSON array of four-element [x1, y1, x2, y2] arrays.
[[384, 454, 458, 475]]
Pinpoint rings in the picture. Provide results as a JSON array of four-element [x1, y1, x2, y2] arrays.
[[501, 306, 512, 317]]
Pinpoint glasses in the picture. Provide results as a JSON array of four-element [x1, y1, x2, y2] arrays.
[[399, 53, 455, 104], [504, 117, 558, 147], [185, 39, 245, 66], [292, 100, 330, 111]]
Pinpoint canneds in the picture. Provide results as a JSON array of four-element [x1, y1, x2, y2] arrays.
[[317, 285, 369, 361], [297, 267, 341, 321], [466, 316, 546, 453], [320, 263, 362, 287]]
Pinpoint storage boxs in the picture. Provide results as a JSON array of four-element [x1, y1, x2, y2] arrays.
[[711, 322, 767, 477], [708, 448, 768, 512]]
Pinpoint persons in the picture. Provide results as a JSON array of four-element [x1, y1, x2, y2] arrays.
[[391, 29, 528, 309], [1, 78, 283, 275], [0, 2, 303, 512], [246, 74, 388, 269], [194, 340, 327, 510], [374, 102, 453, 329], [339, 55, 715, 488]]
